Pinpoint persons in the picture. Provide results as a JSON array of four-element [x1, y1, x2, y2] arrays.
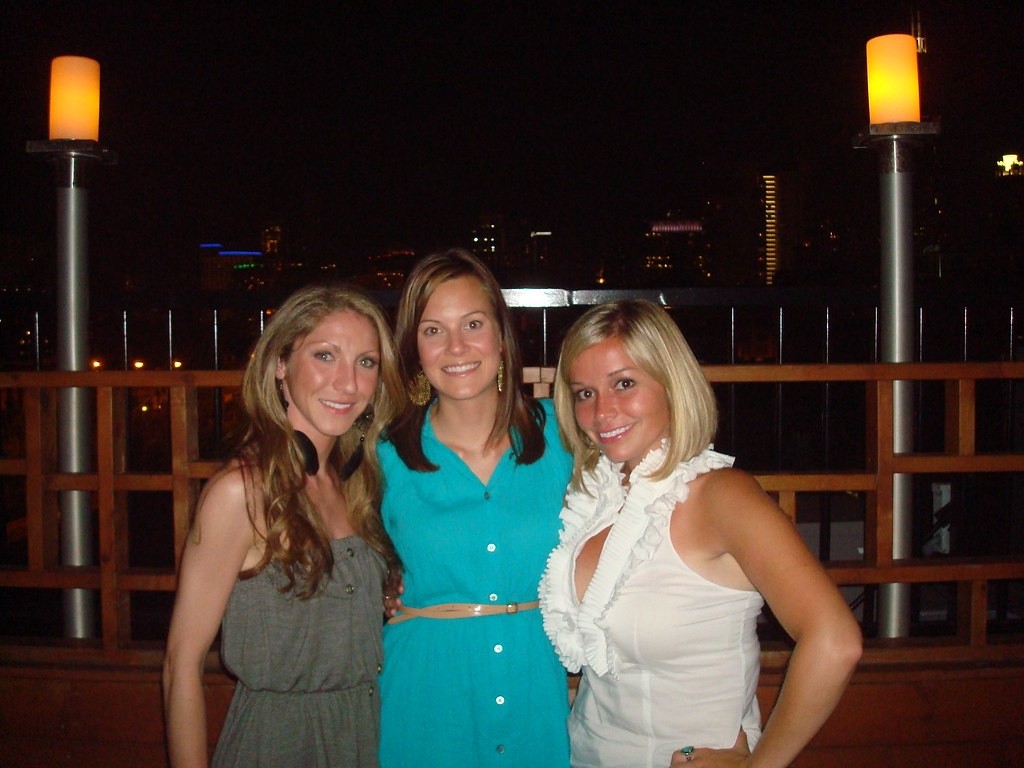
[[161, 290, 405, 768], [386, 300, 862, 767], [377, 247, 580, 768]]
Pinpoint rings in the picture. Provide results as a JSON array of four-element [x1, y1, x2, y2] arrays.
[[682, 746, 696, 761]]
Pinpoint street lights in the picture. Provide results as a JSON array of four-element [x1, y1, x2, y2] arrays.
[[850, 4, 942, 639], [22, 53, 120, 640]]
